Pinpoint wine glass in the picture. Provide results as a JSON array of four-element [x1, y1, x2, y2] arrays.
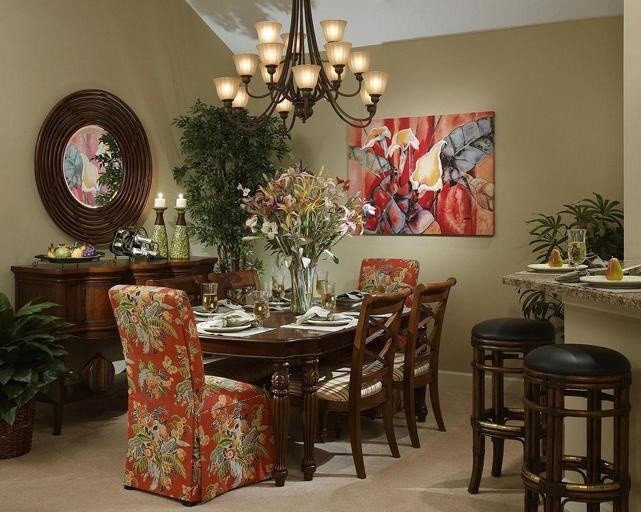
[[567, 228, 588, 277], [252, 272, 287, 327], [317, 270, 336, 317], [201, 281, 218, 323]]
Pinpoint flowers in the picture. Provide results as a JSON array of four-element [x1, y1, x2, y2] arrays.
[[348, 115, 496, 235], [63, 124, 123, 209], [236, 158, 382, 313]]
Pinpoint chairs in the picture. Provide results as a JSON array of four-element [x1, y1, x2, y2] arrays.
[[357, 254, 423, 421], [206, 269, 266, 302], [337, 275, 459, 450], [287, 285, 413, 481], [106, 284, 282, 508], [141, 274, 262, 385]]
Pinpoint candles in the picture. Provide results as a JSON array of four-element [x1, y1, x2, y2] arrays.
[[154, 191, 167, 207], [174, 192, 187, 208]]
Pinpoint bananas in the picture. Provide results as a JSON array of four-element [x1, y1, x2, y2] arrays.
[[47, 243, 55, 258]]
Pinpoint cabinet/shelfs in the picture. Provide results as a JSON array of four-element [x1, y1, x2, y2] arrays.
[[8, 256, 221, 438]]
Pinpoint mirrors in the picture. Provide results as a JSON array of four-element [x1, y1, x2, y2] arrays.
[[33, 88, 156, 247]]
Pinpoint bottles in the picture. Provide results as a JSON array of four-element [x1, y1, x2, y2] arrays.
[[109, 229, 159, 259]]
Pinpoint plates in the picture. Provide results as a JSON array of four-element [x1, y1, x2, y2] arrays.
[[528, 263, 589, 271], [338, 295, 365, 304], [305, 317, 351, 325], [206, 324, 253, 333], [193, 311, 223, 317], [35, 254, 104, 262], [581, 276, 640, 287]]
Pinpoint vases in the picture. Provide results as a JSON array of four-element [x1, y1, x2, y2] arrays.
[[288, 262, 320, 315]]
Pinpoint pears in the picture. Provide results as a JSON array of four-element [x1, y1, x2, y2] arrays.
[[606, 255, 624, 280], [548, 247, 563, 267], [71, 248, 83, 258]]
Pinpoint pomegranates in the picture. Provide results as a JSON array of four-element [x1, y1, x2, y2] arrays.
[[54, 243, 71, 258]]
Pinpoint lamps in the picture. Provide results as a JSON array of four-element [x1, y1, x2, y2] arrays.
[[210, 0, 390, 134]]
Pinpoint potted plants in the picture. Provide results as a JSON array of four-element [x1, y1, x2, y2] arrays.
[[0, 291, 86, 460]]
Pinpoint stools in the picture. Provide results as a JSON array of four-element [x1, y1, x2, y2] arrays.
[[520, 340, 634, 512], [465, 315, 560, 494]]
